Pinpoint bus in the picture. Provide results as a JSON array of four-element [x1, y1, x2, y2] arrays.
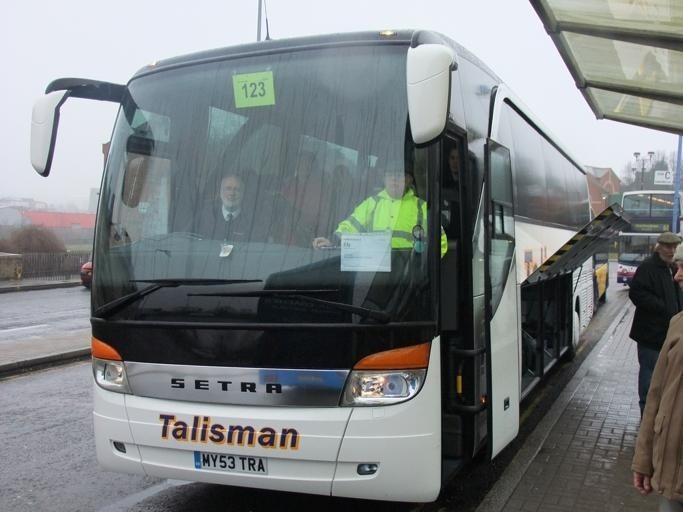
[[29, 28, 631, 503], [617, 189, 683, 288]]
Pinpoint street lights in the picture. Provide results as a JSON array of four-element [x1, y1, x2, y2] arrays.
[[634, 151, 654, 190]]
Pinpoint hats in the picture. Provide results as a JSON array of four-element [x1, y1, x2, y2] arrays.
[[381, 160, 409, 173], [655, 232, 681, 248]]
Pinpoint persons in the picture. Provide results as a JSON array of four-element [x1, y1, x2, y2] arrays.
[[192, 173, 256, 241], [629, 230, 682, 419], [629, 239, 683, 512], [312, 159, 449, 259]]
